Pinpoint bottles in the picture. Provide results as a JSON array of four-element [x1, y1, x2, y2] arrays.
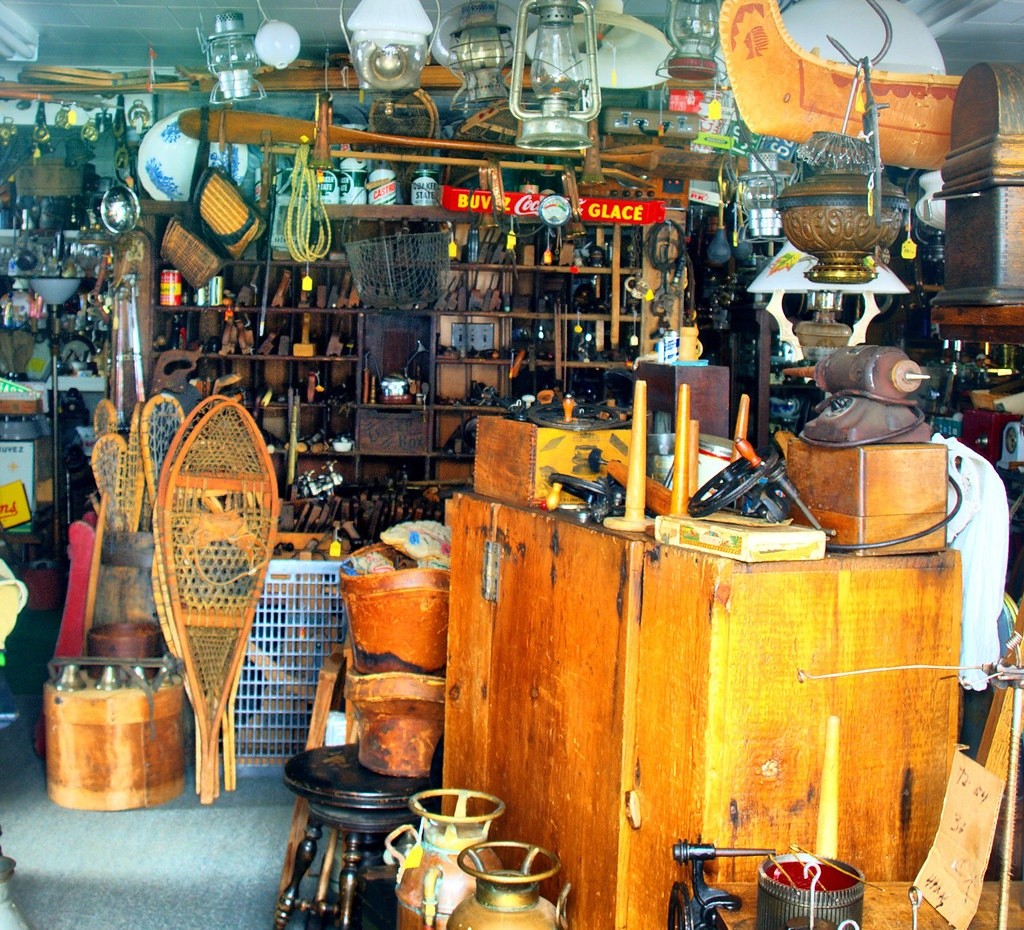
[[159, 269, 182, 307], [562, 394, 574, 423]]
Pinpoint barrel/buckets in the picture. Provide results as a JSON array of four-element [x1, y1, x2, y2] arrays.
[[368, 169, 397, 204], [319, 169, 340, 204], [255, 167, 306, 202], [341, 170, 368, 204], [32, 413, 53, 512], [0, 413, 41, 534], [410, 169, 442, 206]]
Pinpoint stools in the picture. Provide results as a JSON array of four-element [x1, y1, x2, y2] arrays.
[[268, 744, 441, 930]]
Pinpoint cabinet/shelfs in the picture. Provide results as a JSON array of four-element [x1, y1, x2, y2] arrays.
[[0, 227, 106, 394], [107, 202, 688, 506]]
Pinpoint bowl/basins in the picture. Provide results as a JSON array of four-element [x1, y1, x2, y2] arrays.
[[30, 278, 81, 304]]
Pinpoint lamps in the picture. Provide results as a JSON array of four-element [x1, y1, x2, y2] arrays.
[[31, 276, 84, 566], [192, 0, 946, 363]]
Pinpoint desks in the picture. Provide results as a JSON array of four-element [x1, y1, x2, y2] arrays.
[[441, 490, 964, 930]]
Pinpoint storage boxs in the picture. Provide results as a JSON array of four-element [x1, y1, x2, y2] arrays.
[[932, 62, 1024, 306], [476, 414, 634, 510], [15, 158, 95, 198], [785, 437, 950, 559], [933, 416, 962, 437], [965, 409, 1024, 468]]
